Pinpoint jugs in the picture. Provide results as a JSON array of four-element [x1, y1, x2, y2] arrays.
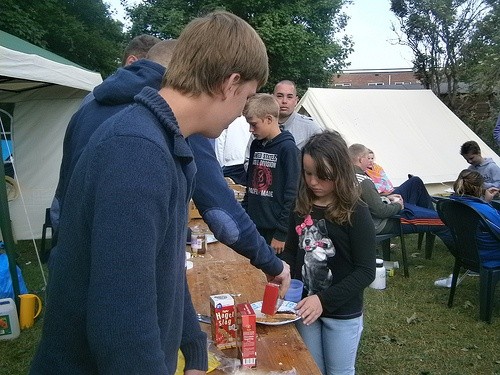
[[17, 294, 42, 329], [369, 258, 386, 289]]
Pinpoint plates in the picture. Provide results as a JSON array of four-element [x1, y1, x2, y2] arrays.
[[186, 233, 217, 244], [250, 301, 301, 326]]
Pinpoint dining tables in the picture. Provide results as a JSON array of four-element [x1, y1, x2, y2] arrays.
[[184, 177, 323, 375]]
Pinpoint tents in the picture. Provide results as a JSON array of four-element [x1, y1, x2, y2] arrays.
[[0, 30, 104, 245], [293, 87, 500, 197]]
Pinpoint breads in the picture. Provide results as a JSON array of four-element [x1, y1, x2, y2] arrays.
[[256, 311, 296, 322]]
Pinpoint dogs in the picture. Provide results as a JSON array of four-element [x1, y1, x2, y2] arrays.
[[295, 214, 336, 297]]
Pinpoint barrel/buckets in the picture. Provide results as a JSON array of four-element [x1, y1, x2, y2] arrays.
[[0, 297, 20, 341]]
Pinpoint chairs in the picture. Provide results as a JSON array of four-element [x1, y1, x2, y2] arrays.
[[41, 208, 53, 247], [436, 199, 500, 324], [408, 174, 438, 260], [375, 215, 410, 277]]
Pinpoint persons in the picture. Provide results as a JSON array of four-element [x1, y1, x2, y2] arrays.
[[449, 169, 500, 268], [349, 144, 448, 235], [50, 38, 291, 298], [242, 93, 301, 279], [28, 11, 269, 374], [365, 148, 395, 197], [244, 80, 322, 172], [281, 129, 376, 375], [78, 35, 162, 109], [460, 141, 500, 200], [493, 112, 500, 146], [215, 116, 256, 187]]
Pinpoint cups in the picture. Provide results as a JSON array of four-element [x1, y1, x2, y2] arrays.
[[284, 279, 303, 302]]
